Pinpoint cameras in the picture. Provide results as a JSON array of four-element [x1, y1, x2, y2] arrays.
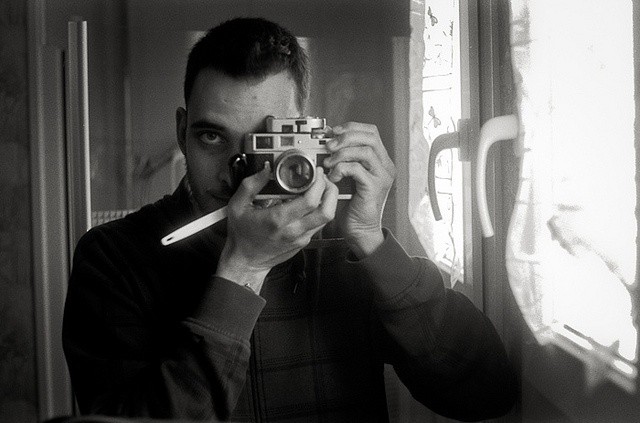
[[233, 114, 356, 200]]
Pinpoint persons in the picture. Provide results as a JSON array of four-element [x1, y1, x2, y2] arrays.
[[59, 13, 516, 421]]
